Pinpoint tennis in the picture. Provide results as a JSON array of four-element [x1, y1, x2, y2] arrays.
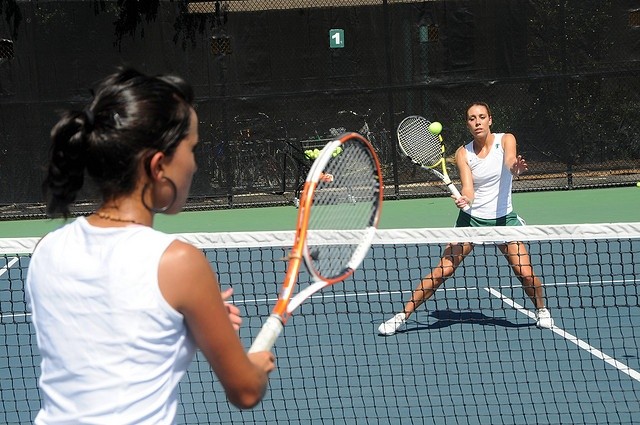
[[429, 122, 443, 134], [304, 147, 343, 160]]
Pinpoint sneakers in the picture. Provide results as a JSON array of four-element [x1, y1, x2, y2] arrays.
[[377, 313, 407, 338], [535, 306, 555, 329]]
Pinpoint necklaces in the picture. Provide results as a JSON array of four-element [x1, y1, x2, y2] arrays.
[[91, 209, 143, 224]]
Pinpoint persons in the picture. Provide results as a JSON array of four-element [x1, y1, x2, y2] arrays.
[[378, 101, 555, 337], [23, 64, 277, 425]]
